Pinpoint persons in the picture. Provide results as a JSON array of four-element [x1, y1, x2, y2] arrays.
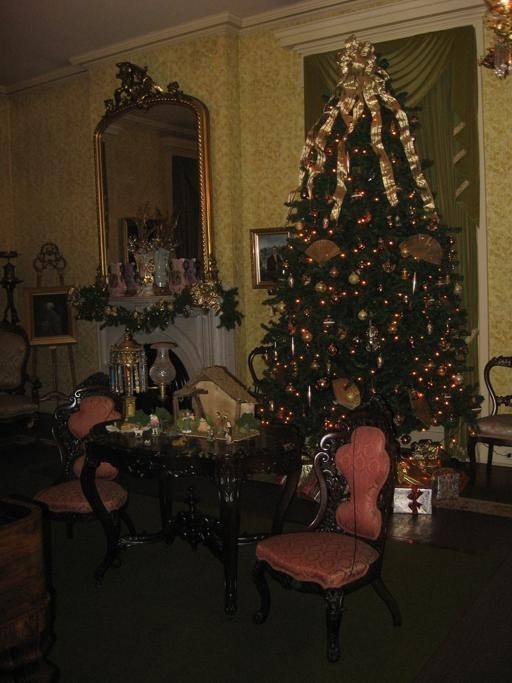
[[39, 300, 63, 333], [265, 245, 285, 281]]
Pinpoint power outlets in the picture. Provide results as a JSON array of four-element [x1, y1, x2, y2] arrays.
[[505, 451, 512, 460]]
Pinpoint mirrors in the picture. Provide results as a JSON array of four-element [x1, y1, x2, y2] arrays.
[[92, 62, 220, 296]]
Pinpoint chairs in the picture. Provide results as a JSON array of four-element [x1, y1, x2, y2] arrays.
[[467, 355, 512, 476], [31, 371, 136, 541], [0, 320, 43, 449], [252, 412, 402, 662]]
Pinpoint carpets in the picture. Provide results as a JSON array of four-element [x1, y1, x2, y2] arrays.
[[1, 438, 503, 683]]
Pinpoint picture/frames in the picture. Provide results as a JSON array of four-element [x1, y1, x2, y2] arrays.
[[121, 215, 163, 272], [249, 226, 292, 289], [23, 286, 79, 346]]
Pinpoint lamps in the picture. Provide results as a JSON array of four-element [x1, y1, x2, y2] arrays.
[[150, 340, 178, 400], [107, 327, 148, 431]]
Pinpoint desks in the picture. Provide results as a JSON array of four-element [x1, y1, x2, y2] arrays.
[[80, 416, 306, 620]]
